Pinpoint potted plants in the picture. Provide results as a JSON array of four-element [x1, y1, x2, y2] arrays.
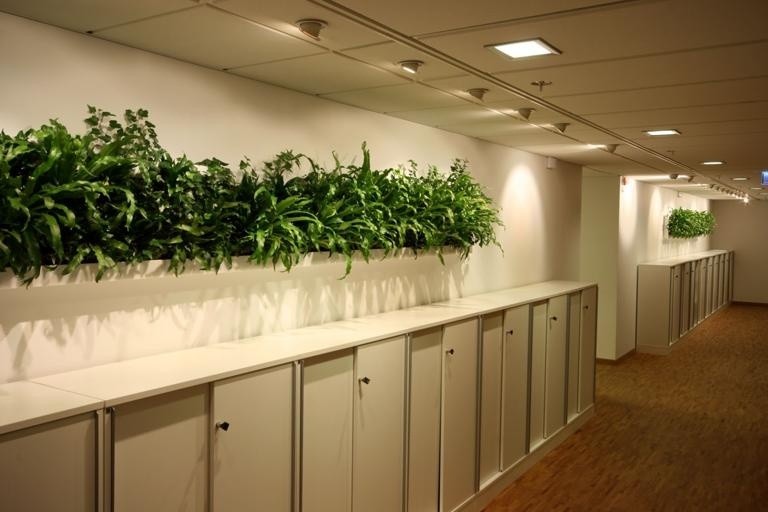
[[0, 104, 506, 290], [663, 206, 718, 241]]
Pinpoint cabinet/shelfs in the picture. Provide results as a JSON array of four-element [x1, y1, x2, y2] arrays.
[[634, 250, 735, 356]]
